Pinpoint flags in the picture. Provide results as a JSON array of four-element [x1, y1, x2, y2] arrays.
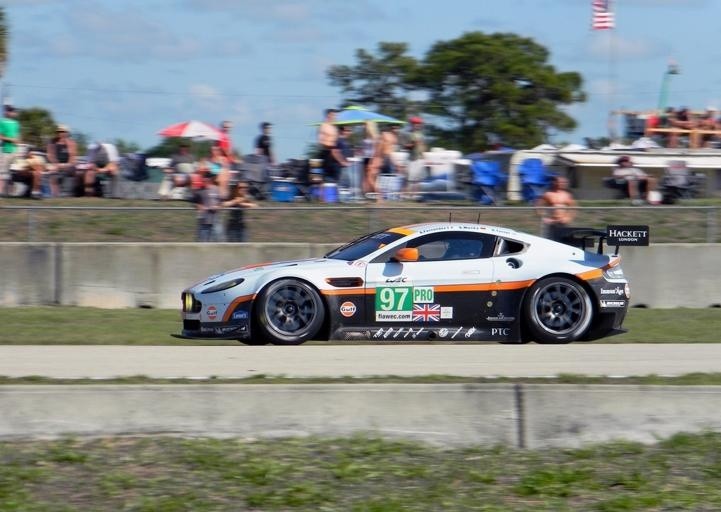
[[591, 0, 615, 30]]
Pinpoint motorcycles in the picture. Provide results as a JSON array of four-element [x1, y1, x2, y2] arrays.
[[662, 160, 706, 204]]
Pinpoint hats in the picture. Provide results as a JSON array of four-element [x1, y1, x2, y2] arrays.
[[55, 124, 69, 132], [408, 117, 423, 123], [86, 141, 100, 154]]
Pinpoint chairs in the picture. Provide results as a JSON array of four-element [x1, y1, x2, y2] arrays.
[[1, 130, 708, 212]]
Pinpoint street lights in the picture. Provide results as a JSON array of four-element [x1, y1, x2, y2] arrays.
[[656, 58, 684, 109]]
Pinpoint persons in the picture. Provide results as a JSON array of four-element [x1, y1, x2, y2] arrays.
[[664, 157, 689, 204], [642, 105, 719, 150], [3, 104, 125, 200], [614, 154, 658, 206], [534, 176, 578, 241], [161, 120, 274, 240], [318, 108, 426, 205]]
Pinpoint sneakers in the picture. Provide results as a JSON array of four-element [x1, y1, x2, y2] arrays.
[[0, 185, 97, 200], [631, 193, 661, 206]]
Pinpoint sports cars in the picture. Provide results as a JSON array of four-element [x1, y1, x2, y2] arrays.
[[172, 221, 649, 343]]
